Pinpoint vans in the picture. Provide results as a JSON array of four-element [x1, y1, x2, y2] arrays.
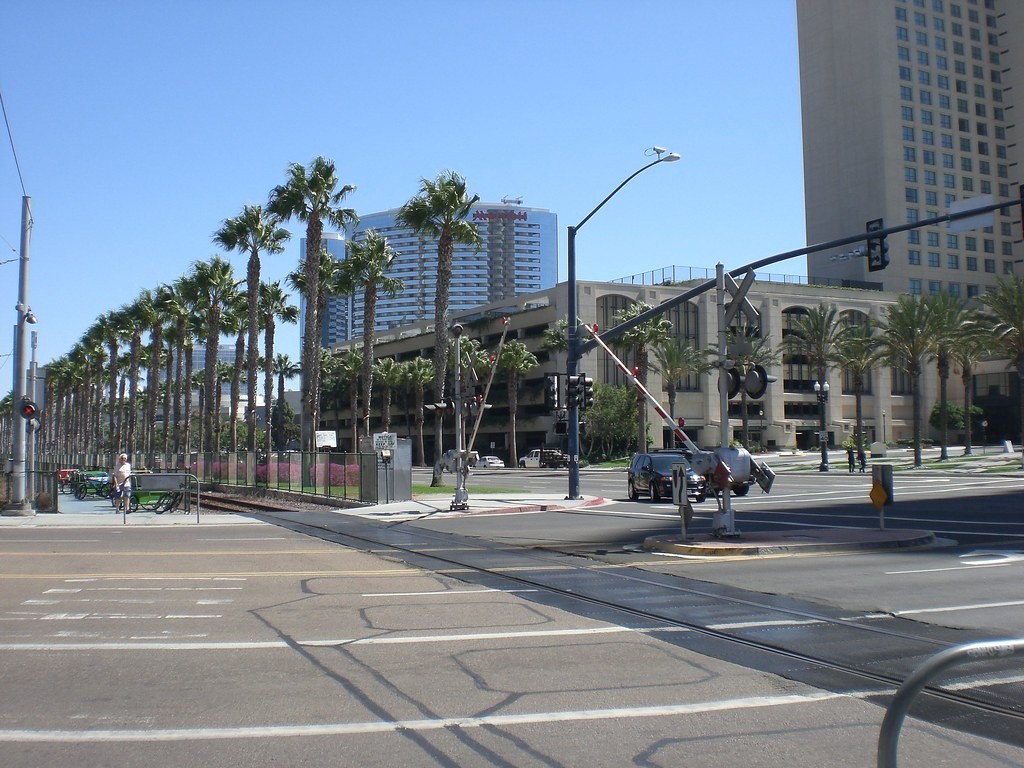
[[443, 449, 480, 471]]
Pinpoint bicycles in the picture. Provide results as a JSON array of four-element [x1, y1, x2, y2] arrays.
[[60, 474, 186, 514]]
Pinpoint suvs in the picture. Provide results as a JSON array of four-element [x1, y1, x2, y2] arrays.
[[626, 448, 749, 503]]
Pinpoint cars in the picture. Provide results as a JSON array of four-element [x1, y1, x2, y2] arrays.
[[475, 456, 505, 469], [519, 449, 568, 468]]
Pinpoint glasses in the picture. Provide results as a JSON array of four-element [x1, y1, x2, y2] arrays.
[[120, 458, 127, 460]]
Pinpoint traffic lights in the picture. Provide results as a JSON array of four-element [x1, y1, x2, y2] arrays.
[[866, 218, 890, 272], [569, 372, 593, 412], [423, 396, 455, 419], [554, 422, 567, 435], [21, 402, 38, 420], [578, 421, 587, 436], [464, 395, 492, 416], [717, 365, 778, 399], [543, 372, 560, 410]]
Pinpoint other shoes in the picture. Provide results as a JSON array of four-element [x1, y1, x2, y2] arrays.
[[126, 509, 130, 514], [116, 511, 120, 514]]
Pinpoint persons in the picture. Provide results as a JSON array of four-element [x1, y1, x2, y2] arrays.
[[113, 454, 132, 514], [857, 449, 866, 473], [848, 448, 857, 473]]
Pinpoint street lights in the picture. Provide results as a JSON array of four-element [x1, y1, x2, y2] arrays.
[[760, 410, 763, 451], [814, 381, 829, 471], [882, 409, 886, 444], [1, 300, 36, 517], [564, 146, 681, 500]]
